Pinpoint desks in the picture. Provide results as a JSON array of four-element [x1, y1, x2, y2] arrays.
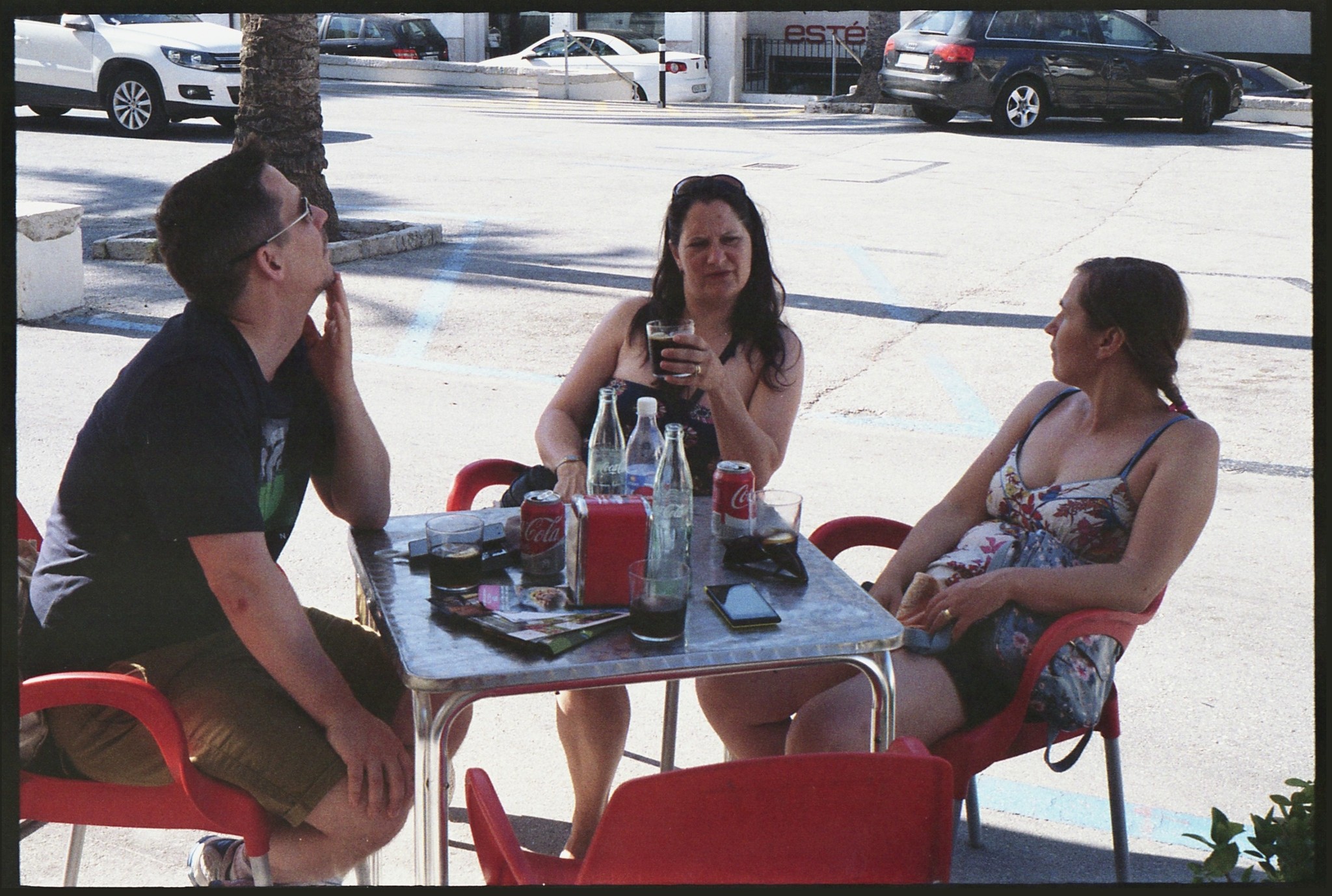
[[346, 496, 905, 886]]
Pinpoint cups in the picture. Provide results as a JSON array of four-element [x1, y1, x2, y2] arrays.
[[646, 318, 695, 381], [748, 488, 803, 553], [425, 513, 485, 598], [627, 558, 692, 648]]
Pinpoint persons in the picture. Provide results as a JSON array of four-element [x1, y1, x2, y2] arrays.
[[534, 174, 806, 860], [697, 258, 1219, 764], [25, 140, 471, 887]]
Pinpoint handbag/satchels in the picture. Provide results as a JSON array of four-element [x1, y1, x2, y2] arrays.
[[971, 530, 1121, 772]]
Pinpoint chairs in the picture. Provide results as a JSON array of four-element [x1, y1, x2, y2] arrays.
[[809, 516, 1168, 882], [466, 735, 952, 886], [18, 497, 274, 888], [1065, 14, 1084, 30], [447, 459, 681, 775]]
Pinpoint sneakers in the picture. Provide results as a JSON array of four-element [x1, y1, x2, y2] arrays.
[[187, 835, 244, 888]]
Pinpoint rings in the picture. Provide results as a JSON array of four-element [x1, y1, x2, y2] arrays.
[[696, 365, 702, 373], [944, 609, 953, 621]]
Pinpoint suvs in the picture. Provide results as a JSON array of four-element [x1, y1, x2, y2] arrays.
[[316, 13, 449, 61], [15, 14, 243, 138]]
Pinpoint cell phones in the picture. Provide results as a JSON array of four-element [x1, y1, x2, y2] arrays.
[[703, 582, 782, 630], [408, 522, 506, 560]]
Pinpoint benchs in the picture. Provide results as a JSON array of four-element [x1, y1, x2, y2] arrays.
[[16, 199, 84, 321]]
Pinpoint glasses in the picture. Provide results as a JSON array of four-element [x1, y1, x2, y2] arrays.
[[673, 174, 747, 198], [228, 196, 314, 263], [722, 528, 809, 587]]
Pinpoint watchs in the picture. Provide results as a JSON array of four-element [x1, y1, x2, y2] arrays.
[[553, 456, 584, 476]]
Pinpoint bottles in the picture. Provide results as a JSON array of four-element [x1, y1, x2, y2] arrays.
[[585, 386, 626, 495], [638, 422, 695, 607], [624, 396, 665, 504]]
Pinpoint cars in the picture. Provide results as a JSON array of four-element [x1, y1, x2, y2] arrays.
[[479, 29, 711, 101], [877, 11, 1243, 136], [1224, 58, 1312, 99]]
